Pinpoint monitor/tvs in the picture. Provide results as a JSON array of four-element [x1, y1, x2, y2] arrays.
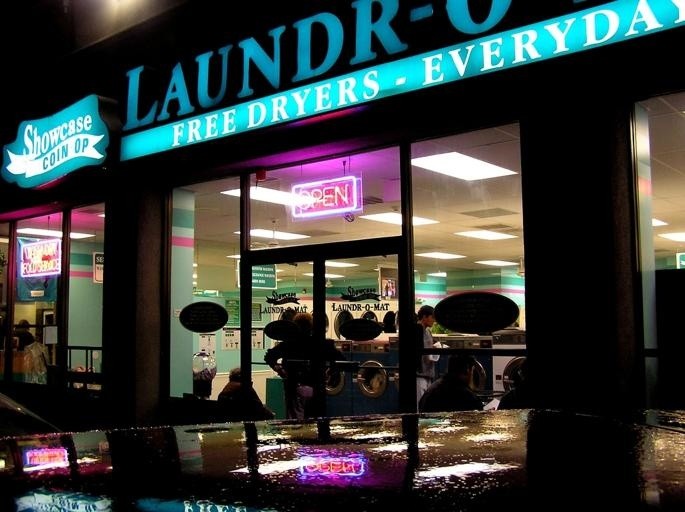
[[381, 278, 398, 299]]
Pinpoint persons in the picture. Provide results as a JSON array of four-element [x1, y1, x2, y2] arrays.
[[417, 355, 484, 411], [414, 304, 438, 413], [262, 312, 317, 420], [487, 360, 528, 409], [218, 368, 273, 424], [13, 319, 36, 352]]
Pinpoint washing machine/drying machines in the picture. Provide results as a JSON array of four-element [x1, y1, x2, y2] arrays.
[[323, 335, 526, 416]]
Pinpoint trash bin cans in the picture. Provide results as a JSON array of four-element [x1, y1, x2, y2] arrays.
[[266, 379, 285, 419]]
